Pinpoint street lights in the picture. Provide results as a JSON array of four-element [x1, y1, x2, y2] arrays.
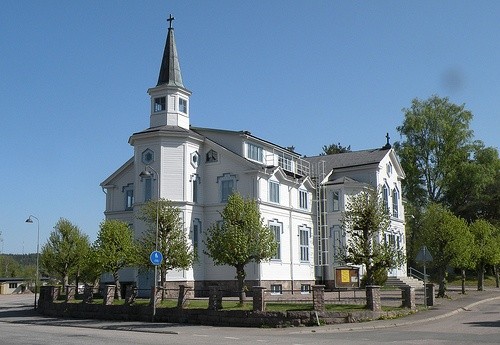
[[26, 215, 41, 310], [140, 166, 160, 322]]
